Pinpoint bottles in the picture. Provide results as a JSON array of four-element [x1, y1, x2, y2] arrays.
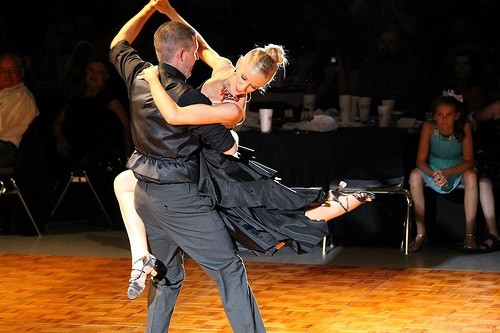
[[378, 106, 391, 126]]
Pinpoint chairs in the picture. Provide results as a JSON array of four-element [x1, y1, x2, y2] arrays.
[[323, 127, 414, 256], [0, 141, 113, 235]]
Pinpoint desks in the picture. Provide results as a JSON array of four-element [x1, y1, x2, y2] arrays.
[[239, 126, 421, 247]]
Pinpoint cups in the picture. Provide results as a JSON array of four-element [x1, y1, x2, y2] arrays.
[[303, 95, 316, 112], [339, 95, 371, 123], [382, 100, 394, 112], [258, 108, 273, 132]]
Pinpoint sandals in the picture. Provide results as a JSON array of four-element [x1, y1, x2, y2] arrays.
[[326, 178, 377, 213], [478, 234, 500, 252], [127, 254, 159, 300], [408, 233, 428, 252], [462, 233, 476, 250]]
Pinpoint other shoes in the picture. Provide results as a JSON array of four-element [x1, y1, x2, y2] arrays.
[[20, 221, 45, 236]]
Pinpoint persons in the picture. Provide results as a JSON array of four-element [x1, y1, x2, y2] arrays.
[[114, 0, 376, 300], [443, 44, 483, 114], [0, 48, 40, 149], [22, 15, 133, 172], [294, 0, 408, 105], [109, 0, 266, 333], [408, 90, 500, 252]]
